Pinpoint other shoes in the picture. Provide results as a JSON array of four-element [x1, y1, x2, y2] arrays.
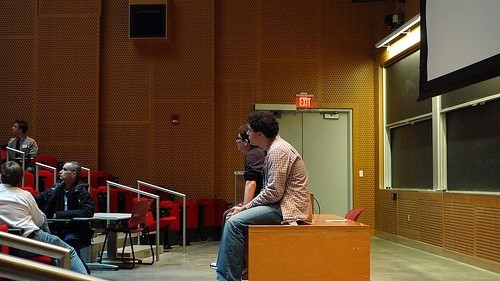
[[242, 268, 247, 275], [210, 263, 218, 269]]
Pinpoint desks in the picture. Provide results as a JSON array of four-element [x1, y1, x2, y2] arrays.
[[73, 213, 131, 221], [248, 214, 370, 281]]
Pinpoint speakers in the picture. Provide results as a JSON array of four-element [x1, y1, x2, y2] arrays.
[[129, 0, 167, 40]]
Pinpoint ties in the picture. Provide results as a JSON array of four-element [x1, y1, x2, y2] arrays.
[[14, 140, 20, 163]]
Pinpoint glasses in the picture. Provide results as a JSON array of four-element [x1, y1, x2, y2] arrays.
[[235, 134, 244, 140]]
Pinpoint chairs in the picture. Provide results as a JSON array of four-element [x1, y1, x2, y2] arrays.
[[345, 208, 364, 222], [0, 150, 227, 270]]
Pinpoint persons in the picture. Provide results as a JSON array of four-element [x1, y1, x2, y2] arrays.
[[216, 110, 311, 281], [43, 160, 96, 274], [0, 160, 88, 275], [209, 124, 267, 271], [6, 120, 38, 175]]
